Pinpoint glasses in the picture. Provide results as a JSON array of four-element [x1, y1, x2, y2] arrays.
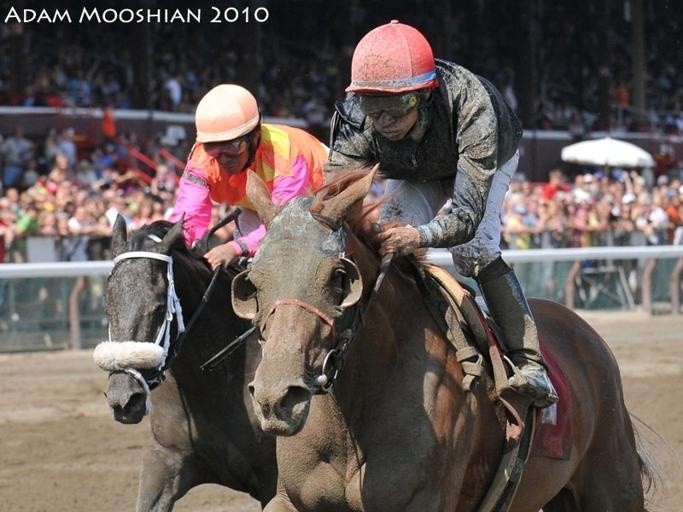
[[354, 88, 425, 120], [201, 135, 251, 159]]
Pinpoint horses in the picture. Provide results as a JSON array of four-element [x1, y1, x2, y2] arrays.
[[102, 213, 279, 512], [245, 161, 661, 512]]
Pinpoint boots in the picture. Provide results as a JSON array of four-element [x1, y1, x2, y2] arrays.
[[474, 267, 560, 410]]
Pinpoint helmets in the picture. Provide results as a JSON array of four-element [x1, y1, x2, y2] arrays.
[[191, 81, 262, 145], [343, 18, 441, 97]]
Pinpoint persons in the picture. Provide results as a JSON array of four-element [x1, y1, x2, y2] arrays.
[[0, 1, 682, 324], [317, 20, 563, 410]]
